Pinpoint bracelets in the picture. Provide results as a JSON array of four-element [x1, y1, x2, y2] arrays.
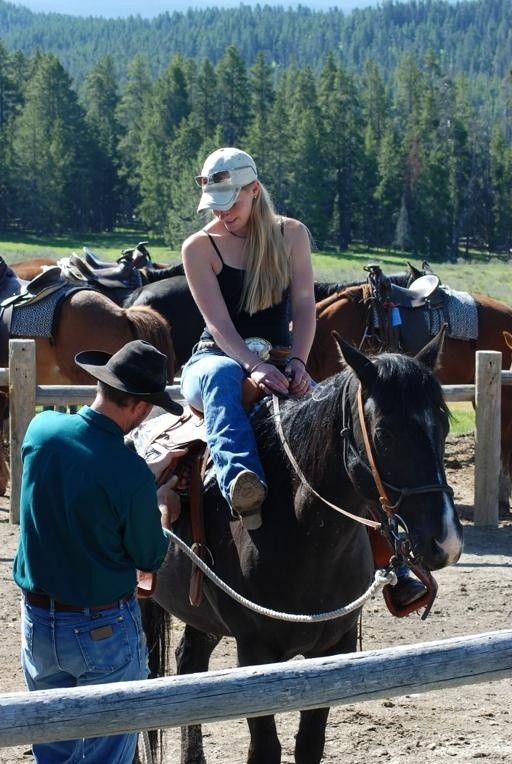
[[249, 359, 264, 372], [288, 357, 306, 366]]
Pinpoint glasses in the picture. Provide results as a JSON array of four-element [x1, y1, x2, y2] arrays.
[[194, 166, 258, 186]]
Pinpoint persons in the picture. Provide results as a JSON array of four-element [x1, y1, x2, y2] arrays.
[[12, 381, 189, 764], [180, 148, 317, 530]]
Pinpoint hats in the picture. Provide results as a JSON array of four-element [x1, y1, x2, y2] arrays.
[[73, 339, 183, 416], [197, 147, 257, 214]]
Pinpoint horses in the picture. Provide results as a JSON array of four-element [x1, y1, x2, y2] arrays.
[[120, 320, 466, 763], [305, 257, 510, 519], [1, 259, 173, 496], [2, 256, 207, 371]]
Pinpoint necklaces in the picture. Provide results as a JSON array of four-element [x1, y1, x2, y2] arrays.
[[223, 225, 248, 238]]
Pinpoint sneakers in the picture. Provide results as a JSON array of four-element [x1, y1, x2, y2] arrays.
[[230, 470, 266, 530], [386, 570, 428, 608]]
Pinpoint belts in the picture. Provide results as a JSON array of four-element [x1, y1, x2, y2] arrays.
[[28, 593, 132, 612]]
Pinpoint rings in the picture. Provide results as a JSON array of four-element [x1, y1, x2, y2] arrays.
[[301, 380, 307, 384]]
